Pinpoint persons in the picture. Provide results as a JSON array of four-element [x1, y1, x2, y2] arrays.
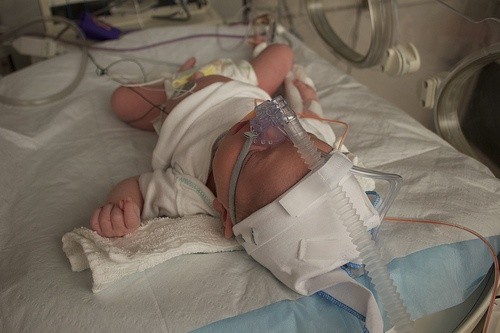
[[90, 32, 380, 278]]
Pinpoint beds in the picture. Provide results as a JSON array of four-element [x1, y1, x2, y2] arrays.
[[1, 22, 500, 333]]
